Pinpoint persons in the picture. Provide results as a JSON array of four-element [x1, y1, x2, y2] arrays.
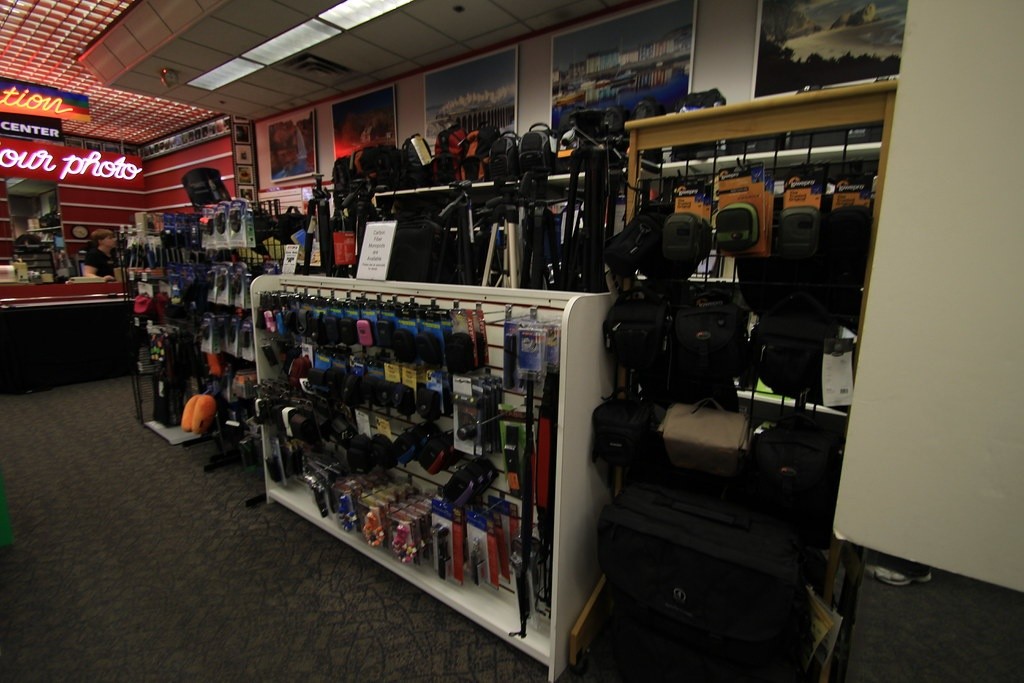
[[874, 552, 933, 588], [82, 227, 121, 283]]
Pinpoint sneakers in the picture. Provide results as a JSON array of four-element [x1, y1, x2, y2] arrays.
[[874, 566, 931, 585]]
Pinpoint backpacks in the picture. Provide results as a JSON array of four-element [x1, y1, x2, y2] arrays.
[[433, 88, 727, 186], [332, 133, 434, 194]]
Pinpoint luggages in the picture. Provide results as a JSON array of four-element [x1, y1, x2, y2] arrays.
[[597, 482, 808, 642]]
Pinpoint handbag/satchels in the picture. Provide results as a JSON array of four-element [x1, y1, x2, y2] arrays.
[[757, 413, 830, 491], [590, 387, 650, 468], [604, 199, 674, 276], [658, 397, 753, 476], [603, 286, 838, 396]]
